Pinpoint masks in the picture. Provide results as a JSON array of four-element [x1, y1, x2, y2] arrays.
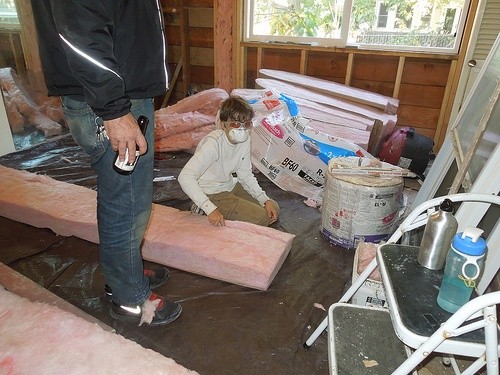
[[229, 126, 249, 143]]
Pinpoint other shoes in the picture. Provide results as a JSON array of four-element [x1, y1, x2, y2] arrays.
[[190, 203, 207, 216]]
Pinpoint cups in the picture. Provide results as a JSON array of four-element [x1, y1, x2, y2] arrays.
[[436, 226, 489, 314]]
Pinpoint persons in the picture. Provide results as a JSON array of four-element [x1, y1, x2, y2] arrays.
[[28, 1, 183, 329], [178, 95, 281, 227]]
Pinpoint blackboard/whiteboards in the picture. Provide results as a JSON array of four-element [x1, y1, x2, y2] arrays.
[[453, 29, 499, 191]]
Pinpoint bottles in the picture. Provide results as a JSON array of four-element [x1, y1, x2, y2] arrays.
[[417, 198, 458, 271], [113, 116, 149, 176]]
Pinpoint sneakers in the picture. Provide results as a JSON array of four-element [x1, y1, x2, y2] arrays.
[[104, 267, 170, 296], [109, 292, 182, 326]]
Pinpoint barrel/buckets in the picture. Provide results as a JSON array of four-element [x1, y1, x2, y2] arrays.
[[320, 156, 405, 250]]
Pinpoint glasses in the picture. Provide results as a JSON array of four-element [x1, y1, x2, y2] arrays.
[[223, 121, 253, 129]]
